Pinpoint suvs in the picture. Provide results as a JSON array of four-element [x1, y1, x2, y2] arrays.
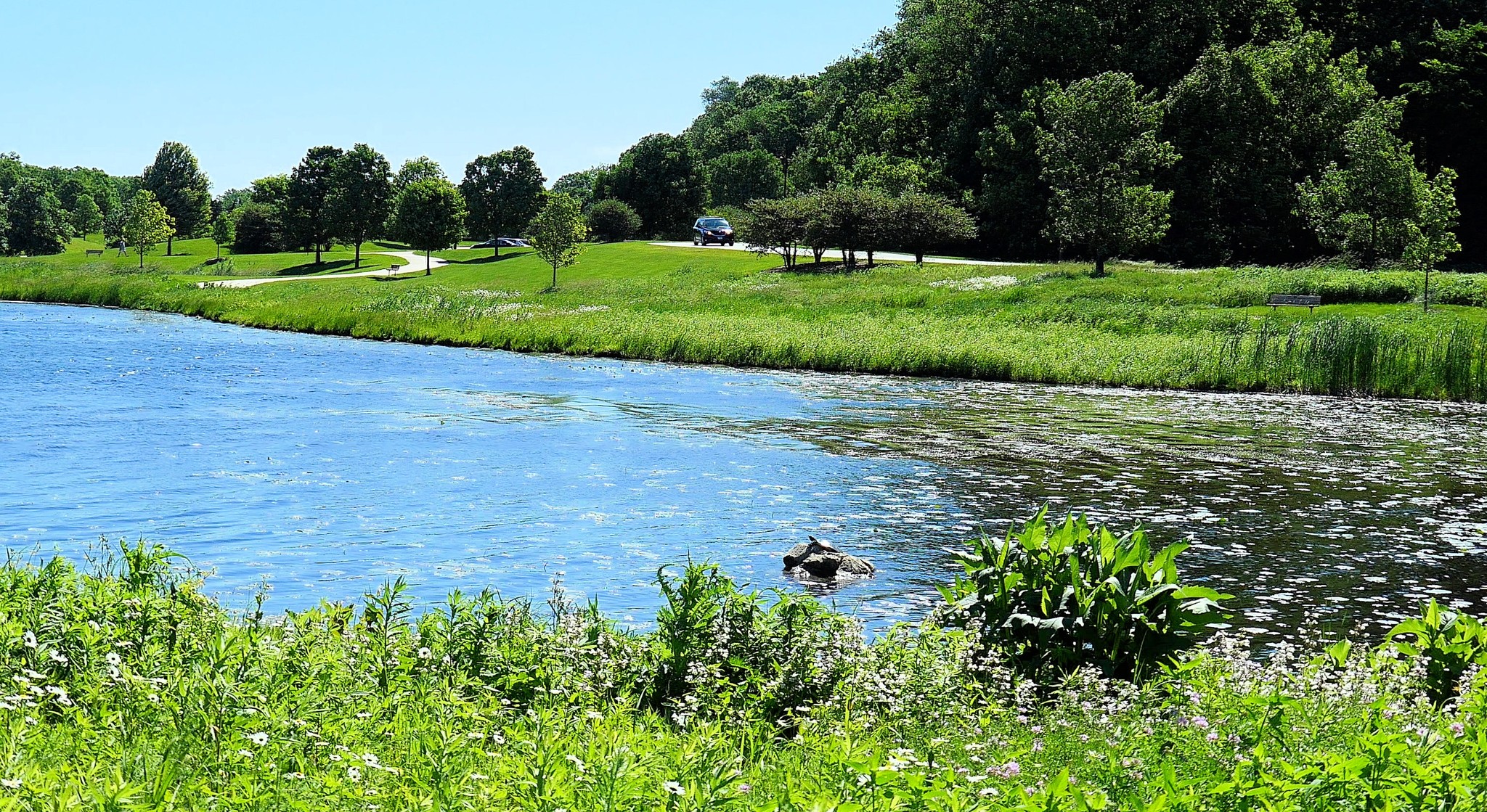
[[692, 217, 735, 246]]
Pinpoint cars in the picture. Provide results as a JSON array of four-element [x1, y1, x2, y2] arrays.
[[470, 237, 533, 249]]
